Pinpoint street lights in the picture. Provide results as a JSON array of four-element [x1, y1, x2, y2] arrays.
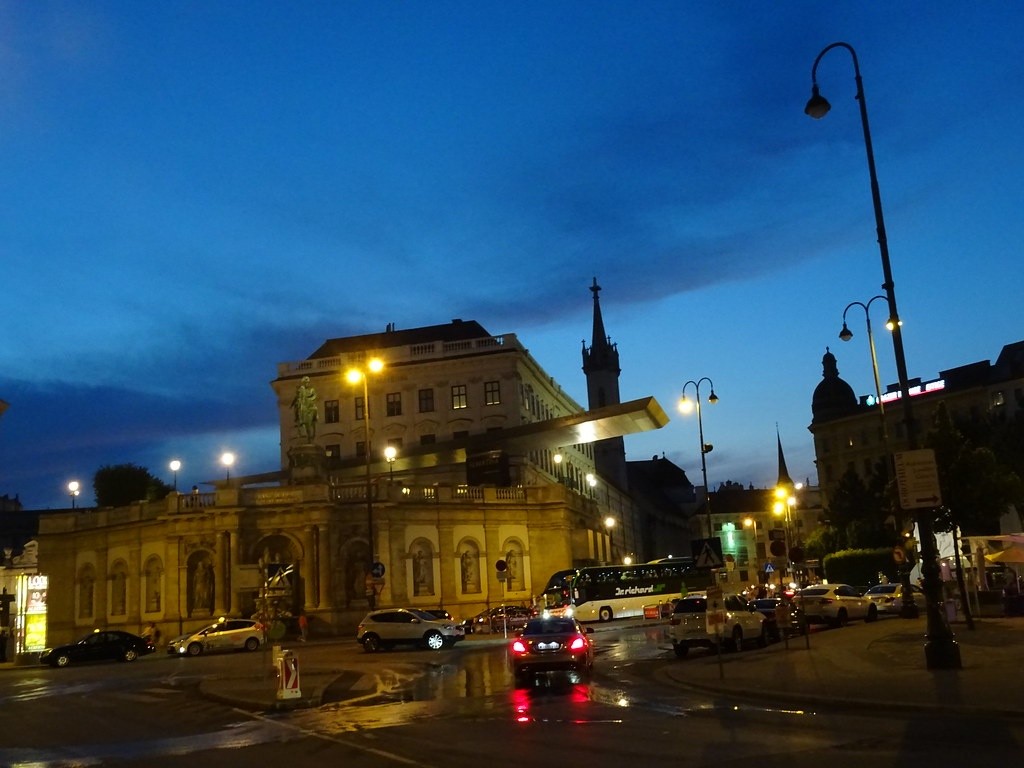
[[680, 378, 719, 569], [840, 294, 922, 621], [805, 42, 965, 672], [744, 482, 802, 592], [345, 359, 384, 612]]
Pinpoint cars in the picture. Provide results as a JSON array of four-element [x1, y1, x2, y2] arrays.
[[757, 599, 809, 639], [792, 583, 878, 627], [38, 630, 157, 668], [508, 617, 595, 678], [166, 619, 269, 657], [462, 605, 540, 633], [861, 583, 926, 616], [422, 609, 467, 629]]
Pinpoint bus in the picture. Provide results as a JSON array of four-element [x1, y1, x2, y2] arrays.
[[535, 557, 717, 622]]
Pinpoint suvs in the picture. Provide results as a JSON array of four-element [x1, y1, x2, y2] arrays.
[[357, 608, 466, 653], [667, 593, 772, 658]]
